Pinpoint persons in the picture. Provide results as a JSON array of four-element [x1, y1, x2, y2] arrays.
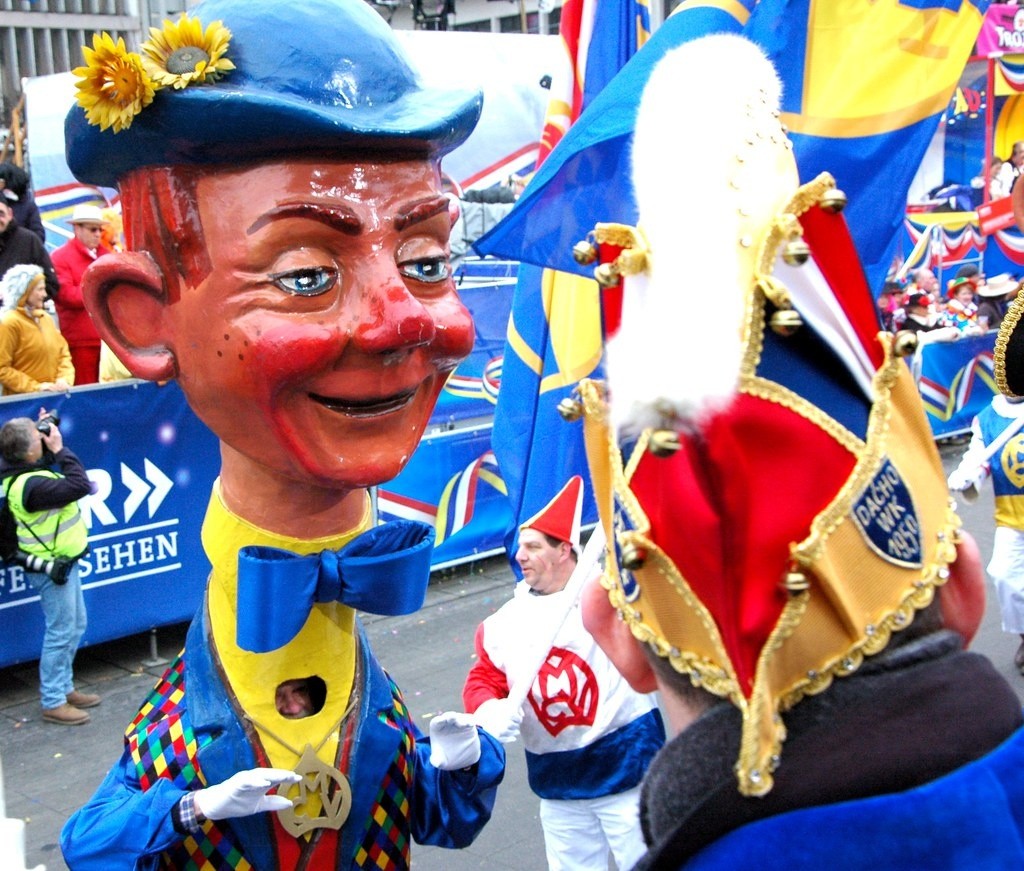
[[585, 35, 1024, 871], [0, 408, 102, 726], [57, 0, 506, 871], [0, 162, 113, 396], [462, 475, 667, 871], [275, 678, 311, 719]]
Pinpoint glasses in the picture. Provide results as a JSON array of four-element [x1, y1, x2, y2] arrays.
[[83, 225, 103, 232]]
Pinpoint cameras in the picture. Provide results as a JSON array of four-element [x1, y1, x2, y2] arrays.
[[13, 550, 72, 585], [35, 415, 61, 437]]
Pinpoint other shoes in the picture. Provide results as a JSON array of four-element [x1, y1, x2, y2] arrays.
[[42, 704, 90, 727], [67, 691, 100, 708]]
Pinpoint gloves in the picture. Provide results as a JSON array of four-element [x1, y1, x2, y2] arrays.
[[194, 768, 303, 820], [470, 699, 523, 744], [429, 712, 482, 770]]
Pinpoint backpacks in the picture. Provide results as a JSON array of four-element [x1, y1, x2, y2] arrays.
[[1, 473, 23, 559]]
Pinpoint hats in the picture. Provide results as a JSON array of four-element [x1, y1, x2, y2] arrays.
[[558, 32, 976, 796], [977, 273, 1019, 297], [946, 276, 975, 299], [63, 205, 111, 225], [516, 475, 583, 546], [900, 293, 933, 307]]
